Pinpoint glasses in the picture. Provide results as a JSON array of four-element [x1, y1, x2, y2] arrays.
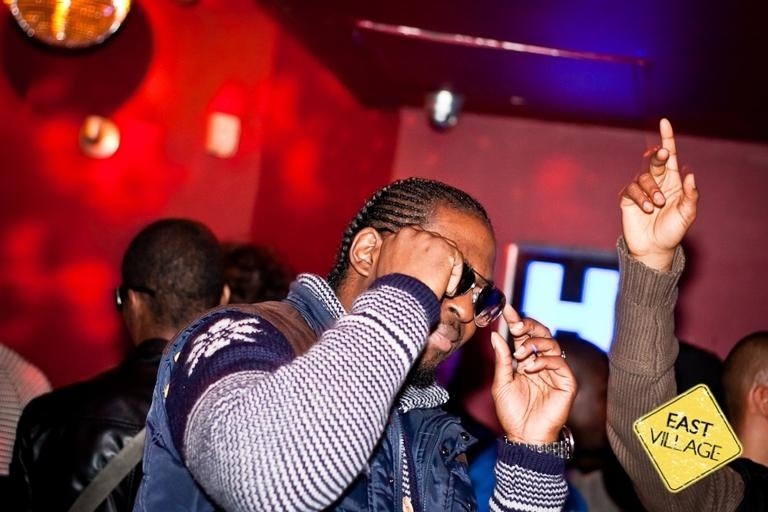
[[113, 282, 157, 308], [377, 226, 508, 330]]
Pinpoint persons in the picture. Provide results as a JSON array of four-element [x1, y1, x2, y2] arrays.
[[0, 117, 768, 510]]
